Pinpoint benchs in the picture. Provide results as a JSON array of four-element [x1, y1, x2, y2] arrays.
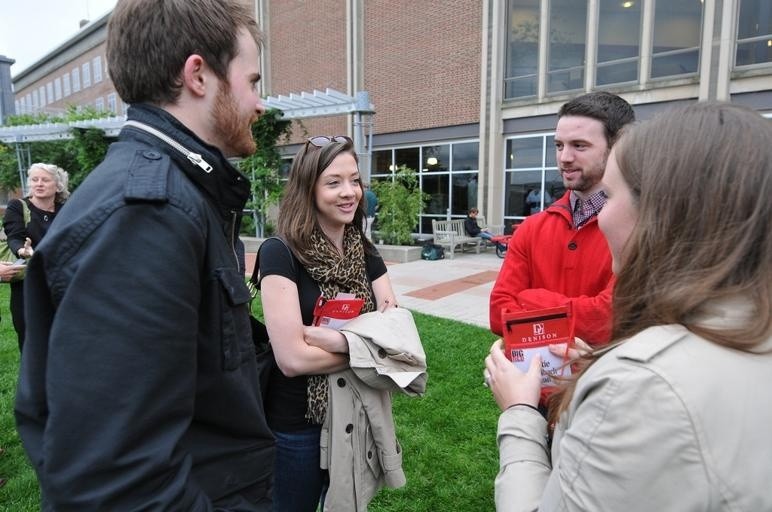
[[432, 216, 505, 260]]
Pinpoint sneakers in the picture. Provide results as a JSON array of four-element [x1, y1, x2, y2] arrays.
[[502, 251, 507, 254]]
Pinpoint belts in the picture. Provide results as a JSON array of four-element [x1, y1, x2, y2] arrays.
[[367, 215, 374, 218]]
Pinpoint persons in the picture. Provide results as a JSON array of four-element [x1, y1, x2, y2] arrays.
[[361, 181, 378, 242], [488, 90, 636, 414], [481, 102, 772, 512], [465, 207, 508, 254], [3, 162, 74, 357], [526, 185, 551, 215], [259, 135, 398, 512], [14, 0, 277, 512], [0, 259, 27, 283]]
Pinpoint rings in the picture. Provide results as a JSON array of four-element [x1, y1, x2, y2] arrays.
[[481, 381, 491, 389]]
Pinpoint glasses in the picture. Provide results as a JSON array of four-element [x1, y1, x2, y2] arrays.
[[296, 136, 354, 180]]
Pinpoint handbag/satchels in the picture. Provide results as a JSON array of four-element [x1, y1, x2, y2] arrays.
[[245, 236, 299, 388], [0, 199, 31, 283]]
